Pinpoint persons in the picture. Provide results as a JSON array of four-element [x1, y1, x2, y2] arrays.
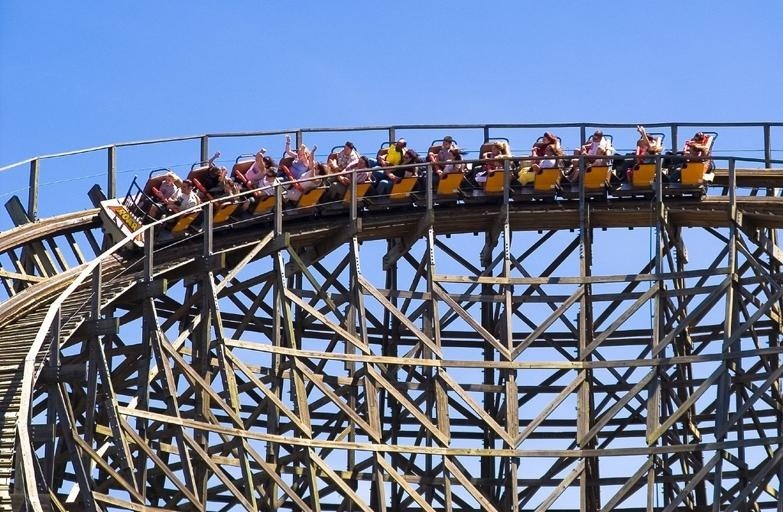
[[133, 123, 707, 234]]
